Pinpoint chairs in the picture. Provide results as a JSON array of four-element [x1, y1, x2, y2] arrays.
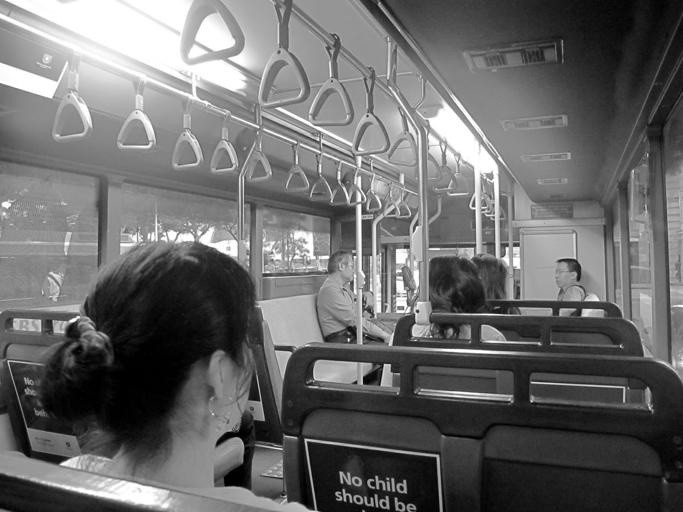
[[0, 292, 281, 512], [281, 292, 683, 511]]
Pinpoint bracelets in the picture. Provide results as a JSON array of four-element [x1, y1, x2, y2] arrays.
[[369, 291, 375, 297]]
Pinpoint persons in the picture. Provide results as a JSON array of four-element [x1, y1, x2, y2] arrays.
[[215, 409, 255, 491], [554, 258, 587, 316], [316, 250, 392, 386], [472, 252, 522, 315], [380, 256, 508, 387], [37, 241, 311, 512]]
